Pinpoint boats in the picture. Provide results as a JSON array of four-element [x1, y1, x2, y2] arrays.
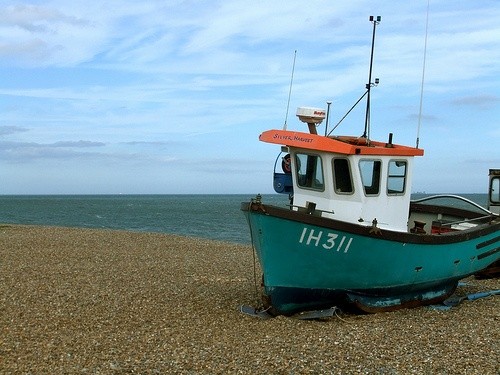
[[241, 16, 498, 315]]
